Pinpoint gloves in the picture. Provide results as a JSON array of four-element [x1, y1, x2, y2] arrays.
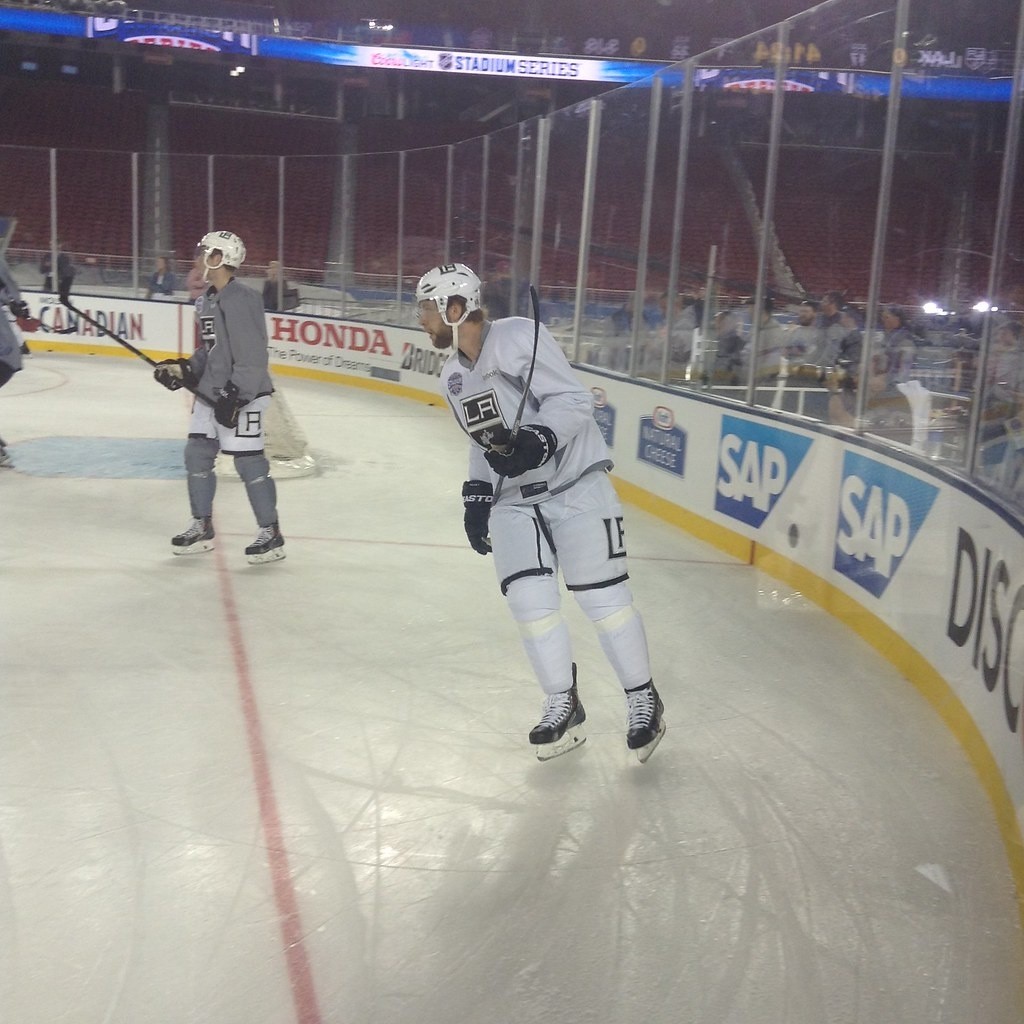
[[11, 299, 29, 318], [483, 424, 558, 478], [214, 381, 250, 429], [154, 358, 198, 391], [462, 478, 494, 555]]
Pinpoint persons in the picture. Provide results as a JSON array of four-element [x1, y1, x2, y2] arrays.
[[40, 241, 68, 291], [262, 262, 286, 310], [612, 286, 1023, 427], [186, 254, 209, 304], [416, 265, 665, 762], [0, 255, 23, 465], [145, 256, 174, 300], [154, 231, 287, 565]]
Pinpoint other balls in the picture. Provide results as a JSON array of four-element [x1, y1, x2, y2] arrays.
[[428, 403, 434, 406]]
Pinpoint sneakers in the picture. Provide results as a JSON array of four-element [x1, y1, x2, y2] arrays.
[[172, 516, 216, 554], [246, 521, 286, 564], [530, 662, 586, 762], [625, 677, 666, 762]]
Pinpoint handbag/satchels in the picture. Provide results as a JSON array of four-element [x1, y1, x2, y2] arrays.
[[281, 289, 301, 311]]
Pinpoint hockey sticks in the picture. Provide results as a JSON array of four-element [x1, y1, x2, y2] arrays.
[[490, 282, 540, 508], [28, 316, 78, 335], [57, 265, 219, 408]]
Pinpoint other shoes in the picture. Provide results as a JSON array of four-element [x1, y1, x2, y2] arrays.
[[0, 446, 15, 468]]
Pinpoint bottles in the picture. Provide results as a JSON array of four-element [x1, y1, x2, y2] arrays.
[[926, 417, 943, 460], [794, 364, 848, 393]]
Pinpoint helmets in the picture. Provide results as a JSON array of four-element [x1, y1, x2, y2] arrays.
[[415, 263, 481, 325], [199, 231, 246, 268]]
